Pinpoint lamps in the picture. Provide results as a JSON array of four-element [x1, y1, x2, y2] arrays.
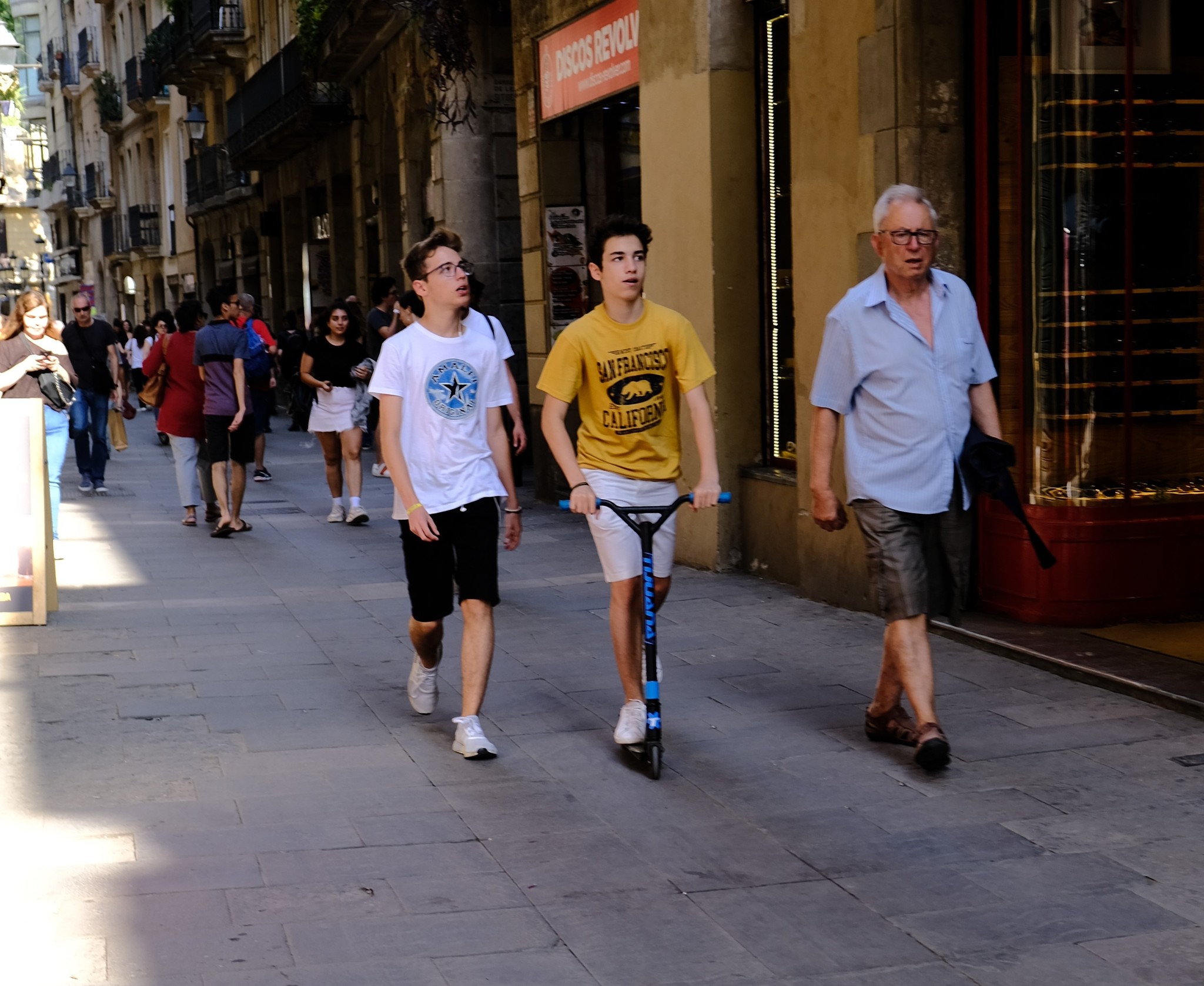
[[25, 170, 42, 190], [311, 73, 368, 122], [183, 106, 227, 160], [59, 163, 89, 200]]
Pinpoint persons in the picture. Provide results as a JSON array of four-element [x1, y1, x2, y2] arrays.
[[367, 226, 525, 760], [809, 184, 1019, 770], [0, 276, 527, 537], [535, 217, 722, 743]]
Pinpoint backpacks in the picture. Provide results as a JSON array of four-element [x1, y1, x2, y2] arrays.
[[239, 318, 276, 387]]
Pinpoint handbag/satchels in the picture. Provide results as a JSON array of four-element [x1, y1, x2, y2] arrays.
[[123, 401, 136, 420], [18, 331, 77, 410], [137, 333, 173, 408], [107, 409, 128, 452], [962, 418, 1059, 568]]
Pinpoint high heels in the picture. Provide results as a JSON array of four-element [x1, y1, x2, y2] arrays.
[[288, 410, 310, 432]]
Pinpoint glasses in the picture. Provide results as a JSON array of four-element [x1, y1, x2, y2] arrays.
[[882, 229, 940, 245], [73, 305, 90, 312], [389, 289, 401, 295], [419, 261, 474, 276], [231, 299, 241, 307]]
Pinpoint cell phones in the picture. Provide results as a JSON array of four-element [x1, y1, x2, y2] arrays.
[[41, 350, 52, 361]]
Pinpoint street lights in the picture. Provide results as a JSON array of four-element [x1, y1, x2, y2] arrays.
[[9, 250, 19, 302], [34, 233, 46, 292]]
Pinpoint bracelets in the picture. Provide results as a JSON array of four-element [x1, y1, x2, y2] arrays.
[[570, 482, 590, 491], [504, 506, 523, 513], [407, 503, 422, 515]]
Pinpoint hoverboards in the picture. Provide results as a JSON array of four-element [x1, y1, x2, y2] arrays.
[[559, 491, 734, 780]]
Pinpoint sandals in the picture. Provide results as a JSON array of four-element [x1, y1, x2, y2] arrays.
[[205, 504, 222, 523], [864, 705, 920, 747], [912, 721, 952, 771], [182, 515, 198, 526]]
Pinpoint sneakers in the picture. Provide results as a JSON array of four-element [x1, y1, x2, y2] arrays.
[[327, 504, 346, 522], [451, 714, 498, 760], [640, 644, 663, 684], [407, 639, 443, 716], [613, 698, 647, 744], [371, 462, 392, 478], [78, 472, 91, 491], [254, 465, 272, 481], [345, 505, 370, 524], [93, 478, 108, 492]]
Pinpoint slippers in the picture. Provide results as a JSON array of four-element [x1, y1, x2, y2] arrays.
[[230, 518, 253, 531], [209, 525, 236, 538]]
